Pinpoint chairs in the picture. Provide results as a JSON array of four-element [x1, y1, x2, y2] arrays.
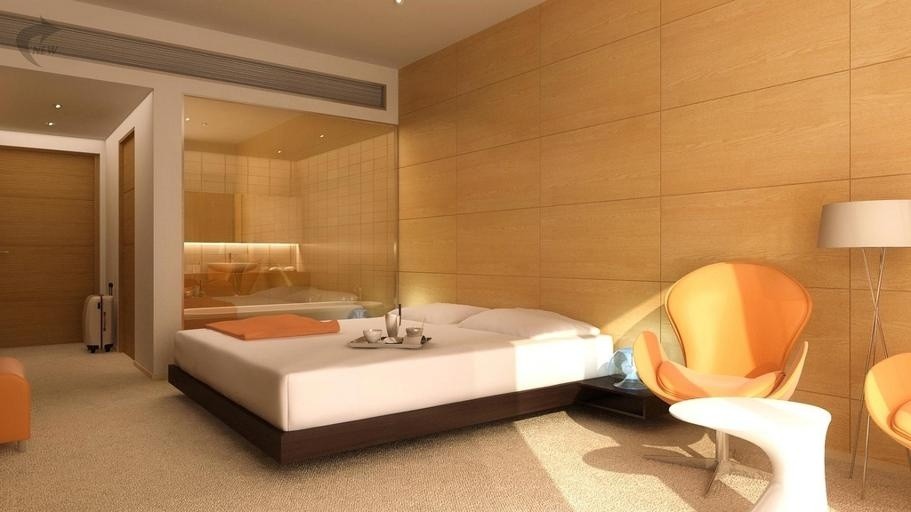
[[633, 261, 813, 499], [864, 352, 911, 450]]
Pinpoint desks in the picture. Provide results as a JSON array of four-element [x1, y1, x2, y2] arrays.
[[668, 397, 832, 512], [576, 374, 672, 420]]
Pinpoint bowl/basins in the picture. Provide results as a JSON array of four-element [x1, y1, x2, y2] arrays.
[[363, 330, 380, 341]]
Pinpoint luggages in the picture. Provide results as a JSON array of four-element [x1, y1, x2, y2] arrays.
[[83, 281, 115, 353]]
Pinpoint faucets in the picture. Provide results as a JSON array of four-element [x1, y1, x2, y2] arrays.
[[228, 252, 231, 263]]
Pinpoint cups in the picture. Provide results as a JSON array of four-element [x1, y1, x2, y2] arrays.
[[386, 314, 400, 338], [406, 328, 422, 336]]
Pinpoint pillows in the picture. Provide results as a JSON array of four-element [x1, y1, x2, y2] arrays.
[[251, 287, 303, 299], [387, 302, 489, 324], [459, 308, 600, 339], [288, 288, 358, 303]]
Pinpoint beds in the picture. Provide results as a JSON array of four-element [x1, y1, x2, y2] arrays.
[[169, 317, 613, 464]]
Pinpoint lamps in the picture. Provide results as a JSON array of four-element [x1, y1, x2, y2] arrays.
[[821, 199, 911, 499], [609, 347, 647, 390]]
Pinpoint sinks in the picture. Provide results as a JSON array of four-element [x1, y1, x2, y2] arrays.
[[207, 262, 258, 272]]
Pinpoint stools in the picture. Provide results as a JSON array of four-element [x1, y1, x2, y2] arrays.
[[0, 356, 30, 452]]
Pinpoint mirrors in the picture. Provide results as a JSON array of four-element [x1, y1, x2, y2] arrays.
[[183, 192, 242, 242]]
